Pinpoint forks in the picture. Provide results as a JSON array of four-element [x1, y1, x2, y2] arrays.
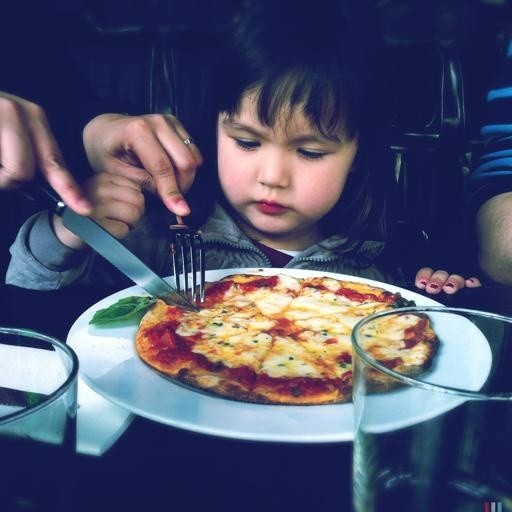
[[170, 213, 208, 304]]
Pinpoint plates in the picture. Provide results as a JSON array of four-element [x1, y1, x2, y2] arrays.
[[65, 266, 495, 445]]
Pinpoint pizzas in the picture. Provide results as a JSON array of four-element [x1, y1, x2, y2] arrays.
[[135, 273, 439, 407]]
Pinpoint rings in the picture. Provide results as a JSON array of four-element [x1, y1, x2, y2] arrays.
[[184, 138, 196, 144]]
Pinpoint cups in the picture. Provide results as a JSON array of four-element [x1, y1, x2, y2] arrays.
[[349, 302, 512, 512], [0, 324, 81, 512]]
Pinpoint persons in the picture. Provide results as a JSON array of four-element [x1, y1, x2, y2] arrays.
[[472, 46, 511, 287], [5, 16, 482, 297], [0, 86, 204, 348]]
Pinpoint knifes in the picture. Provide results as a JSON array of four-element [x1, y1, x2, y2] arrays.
[[18, 178, 202, 314]]
